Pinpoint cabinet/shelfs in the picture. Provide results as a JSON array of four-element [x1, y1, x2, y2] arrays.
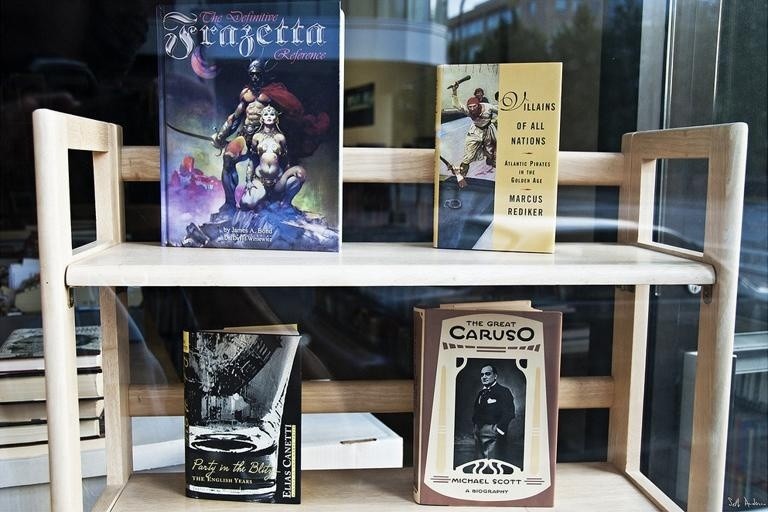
[[32, 109, 744, 512]]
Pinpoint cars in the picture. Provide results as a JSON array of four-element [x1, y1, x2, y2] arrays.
[[299, 286, 489, 381]]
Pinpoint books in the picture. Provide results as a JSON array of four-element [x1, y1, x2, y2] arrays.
[[433, 61, 563, 254], [156, 0, 345, 254], [180, 323, 300, 504], [1, 307, 105, 462], [410, 299, 565, 509]]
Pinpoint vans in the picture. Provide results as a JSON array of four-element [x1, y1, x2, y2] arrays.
[[452, 212, 766, 371]]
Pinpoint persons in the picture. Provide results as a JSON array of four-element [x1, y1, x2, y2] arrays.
[[208, 57, 331, 214], [470, 360, 516, 458], [451, 81, 498, 180], [474, 88, 490, 103], [236, 105, 310, 214]]
